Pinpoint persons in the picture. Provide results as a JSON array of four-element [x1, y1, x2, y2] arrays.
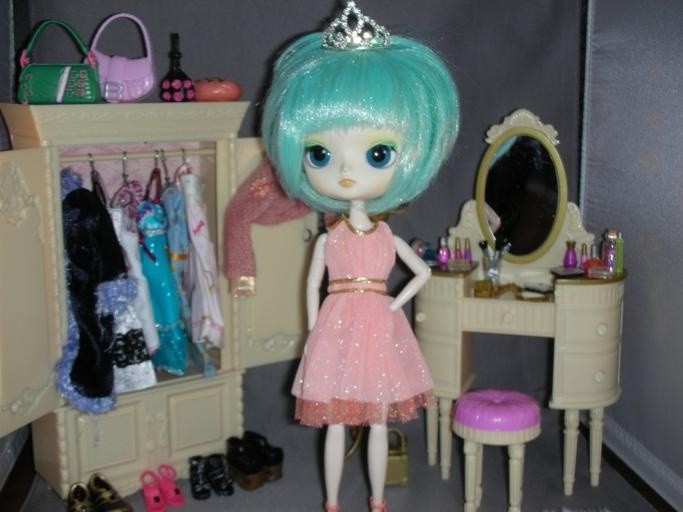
[[259, 0, 462, 512]]
